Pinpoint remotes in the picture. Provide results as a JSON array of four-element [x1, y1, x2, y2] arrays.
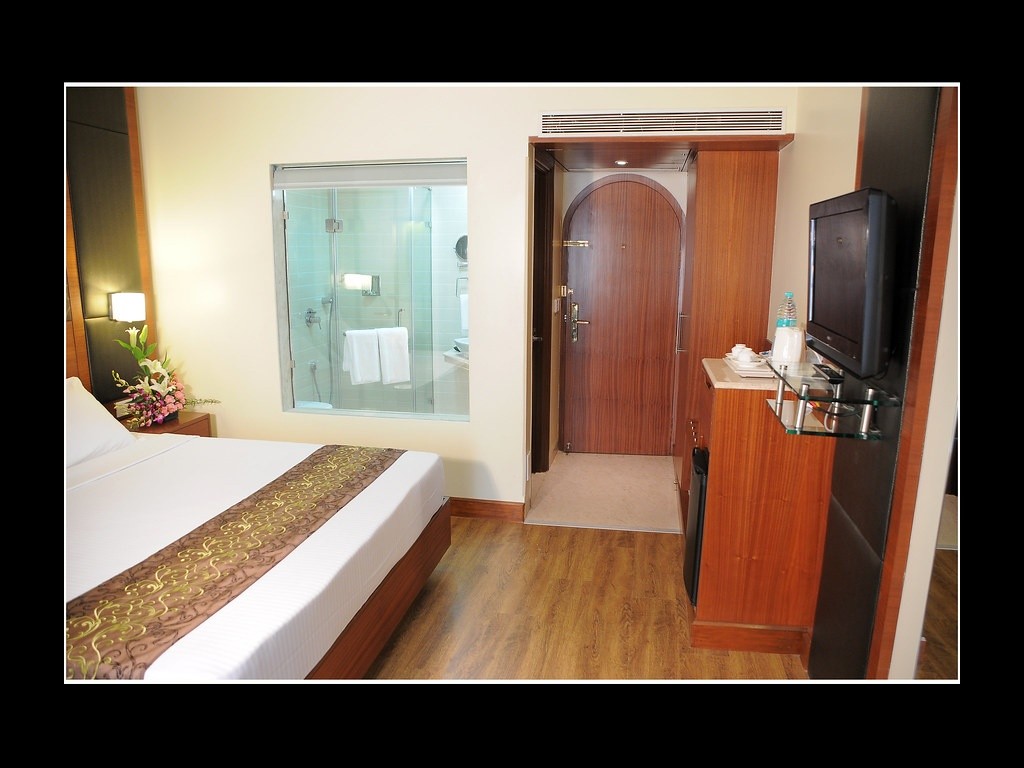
[[812, 363, 844, 384]]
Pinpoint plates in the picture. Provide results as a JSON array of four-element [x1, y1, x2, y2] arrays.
[[732, 362, 771, 373], [731, 358, 767, 368], [727, 353, 760, 359]]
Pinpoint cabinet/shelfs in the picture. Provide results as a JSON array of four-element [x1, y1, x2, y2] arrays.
[[683, 355, 838, 653], [672, 150, 782, 496]]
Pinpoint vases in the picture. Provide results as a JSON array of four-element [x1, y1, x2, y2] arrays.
[[151, 411, 179, 424]]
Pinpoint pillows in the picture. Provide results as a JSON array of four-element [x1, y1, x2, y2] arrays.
[[65, 375, 138, 472]]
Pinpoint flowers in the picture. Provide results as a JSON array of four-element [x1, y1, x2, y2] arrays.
[[111, 325, 222, 429]]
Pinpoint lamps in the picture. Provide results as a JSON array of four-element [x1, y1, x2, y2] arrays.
[[107, 292, 147, 324]]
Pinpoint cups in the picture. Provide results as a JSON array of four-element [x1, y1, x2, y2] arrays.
[[731, 343, 747, 357], [738, 348, 754, 362]]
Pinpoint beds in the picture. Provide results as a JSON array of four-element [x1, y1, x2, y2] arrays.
[[65, 409, 455, 681]]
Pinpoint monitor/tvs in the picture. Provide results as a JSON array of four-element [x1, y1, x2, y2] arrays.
[[805, 188, 896, 378]]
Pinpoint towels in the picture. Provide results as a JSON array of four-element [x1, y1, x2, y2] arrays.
[[342, 329, 382, 386], [458, 293, 469, 331], [375, 326, 411, 386]]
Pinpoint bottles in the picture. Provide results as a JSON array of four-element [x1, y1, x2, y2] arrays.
[[776, 292, 798, 327]]
